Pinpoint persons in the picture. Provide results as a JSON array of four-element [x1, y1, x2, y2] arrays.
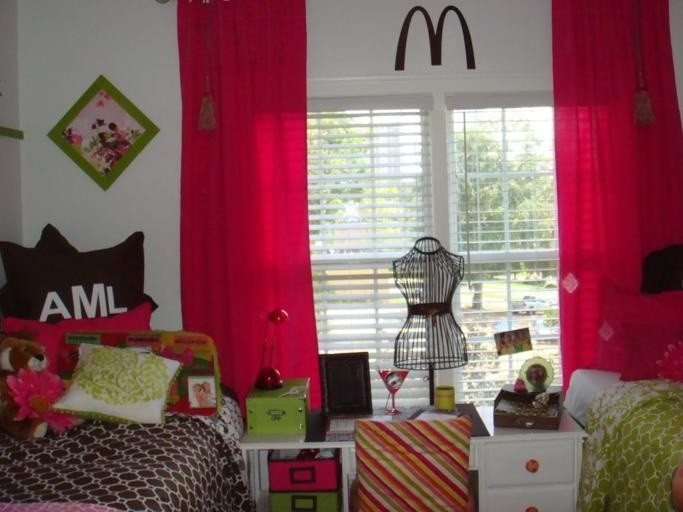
[[192, 381, 215, 407]]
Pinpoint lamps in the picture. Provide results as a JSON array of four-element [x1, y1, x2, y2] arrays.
[[255, 308, 288, 389]]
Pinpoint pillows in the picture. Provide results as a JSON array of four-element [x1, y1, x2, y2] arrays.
[[1, 224, 160, 428], [65, 333, 222, 419], [51, 343, 184, 426]]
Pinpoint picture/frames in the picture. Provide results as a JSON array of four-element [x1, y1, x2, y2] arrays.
[[319, 351, 373, 415]]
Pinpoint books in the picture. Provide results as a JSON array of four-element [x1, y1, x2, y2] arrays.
[[405, 409, 461, 421]]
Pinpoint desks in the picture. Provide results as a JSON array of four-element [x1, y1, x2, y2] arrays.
[[239, 407, 588, 512]]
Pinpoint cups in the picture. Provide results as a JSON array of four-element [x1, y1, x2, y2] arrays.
[[435, 386, 456, 413]]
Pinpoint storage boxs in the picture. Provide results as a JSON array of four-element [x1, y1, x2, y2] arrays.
[[246, 378, 308, 436]]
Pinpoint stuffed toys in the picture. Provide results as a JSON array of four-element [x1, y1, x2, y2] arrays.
[[0, 336, 78, 441]]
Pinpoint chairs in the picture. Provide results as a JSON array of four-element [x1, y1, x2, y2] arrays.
[[348, 416, 478, 512]]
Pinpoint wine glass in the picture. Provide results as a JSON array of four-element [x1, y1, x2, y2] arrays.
[[377, 369, 410, 414]]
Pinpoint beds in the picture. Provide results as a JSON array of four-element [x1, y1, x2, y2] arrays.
[[1, 383, 254, 512], [563, 361, 682, 512]]
